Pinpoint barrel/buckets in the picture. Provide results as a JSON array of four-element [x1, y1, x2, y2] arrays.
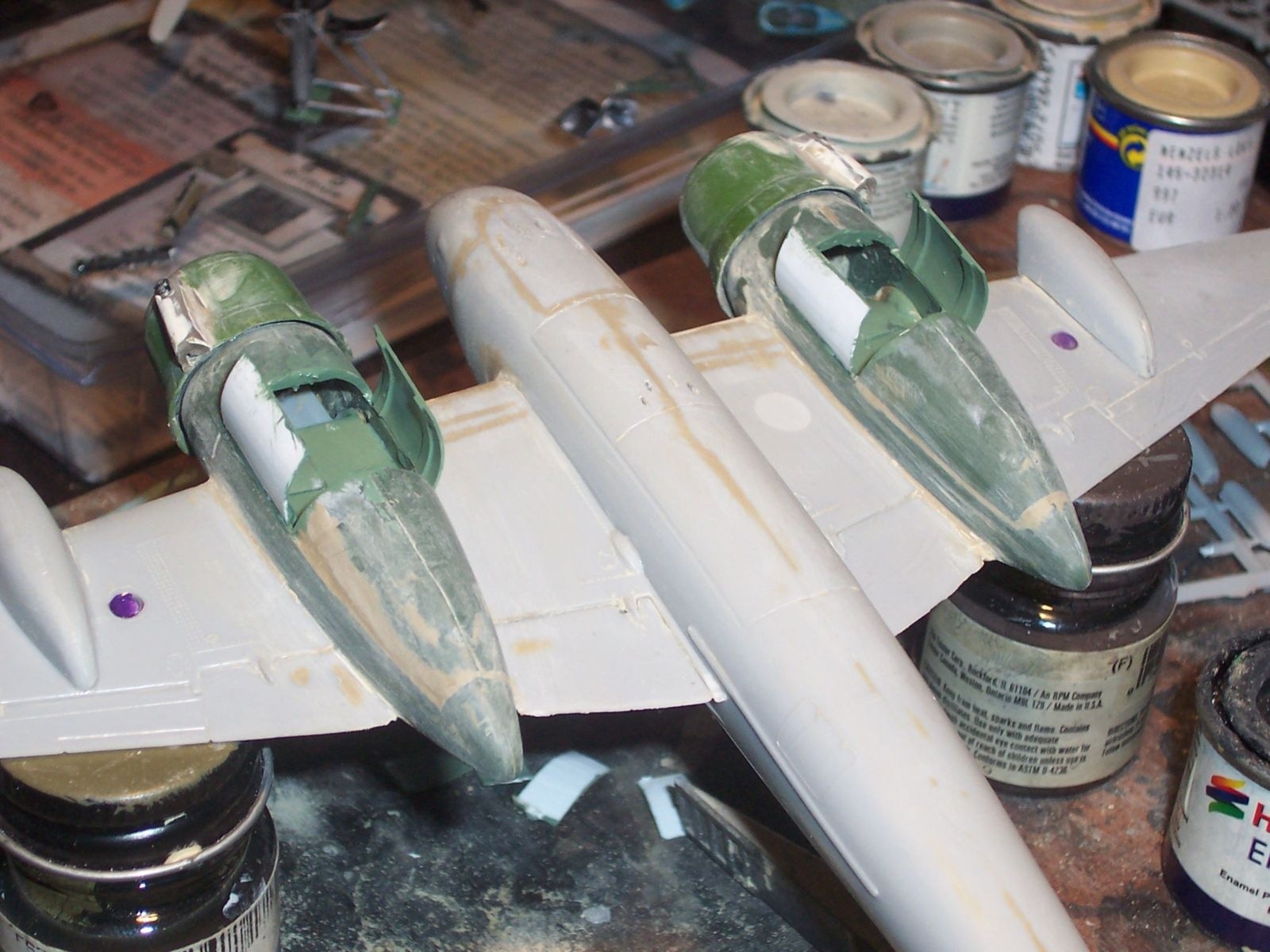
[[991, 0, 1162, 171], [1073, 31, 1270, 251], [740, 59, 942, 245], [856, 0, 1041, 220]]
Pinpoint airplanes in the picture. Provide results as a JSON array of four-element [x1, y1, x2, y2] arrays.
[[0, 131, 1269, 952]]
